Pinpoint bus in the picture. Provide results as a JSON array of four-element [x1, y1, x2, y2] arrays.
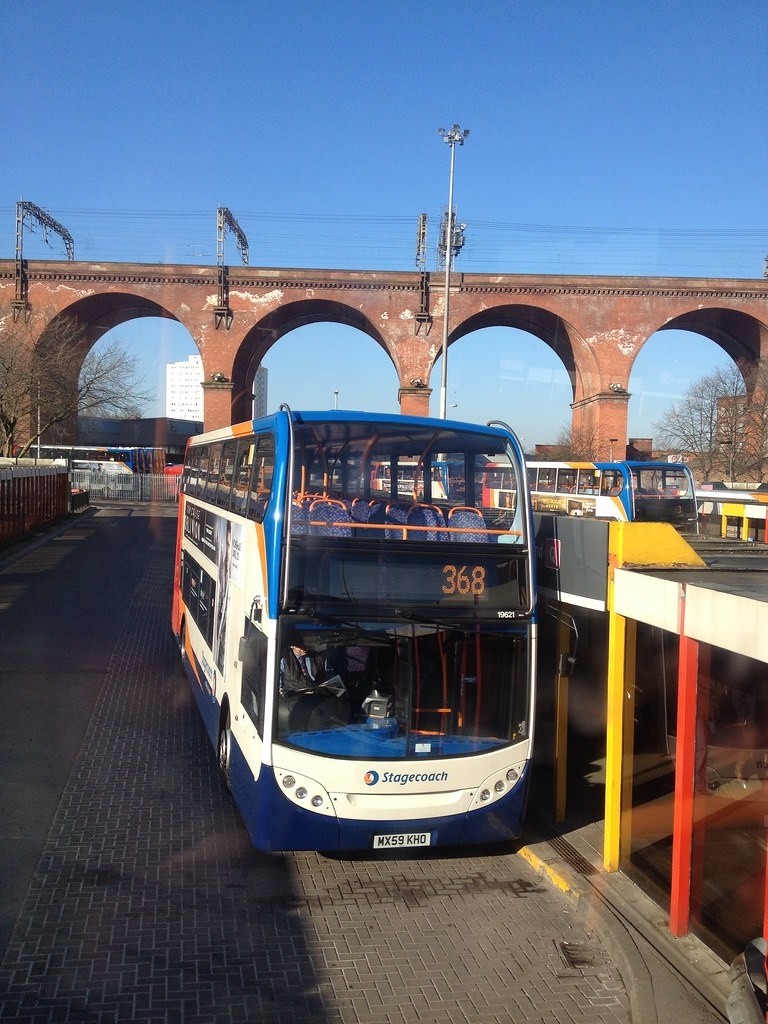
[[15, 444, 167, 499], [360, 460, 484, 504], [480, 460, 699, 531], [172, 403, 579, 858], [667, 486, 768, 544]]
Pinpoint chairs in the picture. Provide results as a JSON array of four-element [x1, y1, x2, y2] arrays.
[[182, 465, 488, 544], [383, 474, 681, 500]]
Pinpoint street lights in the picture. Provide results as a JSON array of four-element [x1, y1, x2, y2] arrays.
[[334, 391, 339, 410], [438, 122, 470, 419]]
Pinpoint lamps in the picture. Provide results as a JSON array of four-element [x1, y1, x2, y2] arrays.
[[610, 383, 625, 393], [211, 373, 229, 383], [410, 379, 427, 387]]
